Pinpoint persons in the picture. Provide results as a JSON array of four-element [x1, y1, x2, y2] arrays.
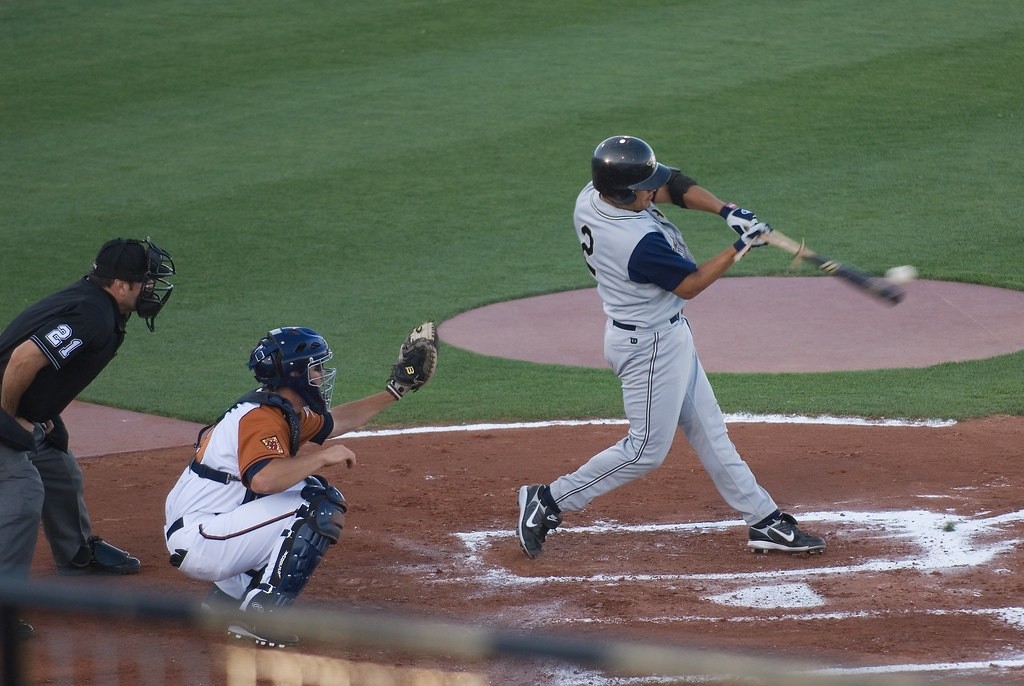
[[164, 319, 440, 649], [518, 134, 825, 556], [0, 240, 179, 636]]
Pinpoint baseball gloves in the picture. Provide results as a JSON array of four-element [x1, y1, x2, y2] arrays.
[[383, 321, 440, 400]]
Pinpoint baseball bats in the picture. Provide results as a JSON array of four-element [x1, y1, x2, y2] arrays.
[[762, 230, 903, 307]]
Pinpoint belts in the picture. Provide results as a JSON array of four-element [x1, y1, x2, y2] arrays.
[[613, 313, 679, 331]]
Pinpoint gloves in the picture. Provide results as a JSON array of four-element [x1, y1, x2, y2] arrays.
[[733, 222, 773, 257], [719, 203, 758, 236]]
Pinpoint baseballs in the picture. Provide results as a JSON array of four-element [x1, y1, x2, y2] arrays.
[[886, 262, 916, 289]]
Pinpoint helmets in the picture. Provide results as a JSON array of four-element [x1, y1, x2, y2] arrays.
[[592, 135, 672, 206], [250, 326, 329, 384], [96, 237, 169, 281]]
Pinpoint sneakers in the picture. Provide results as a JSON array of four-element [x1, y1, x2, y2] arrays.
[[516, 485, 560, 560], [0, 615, 37, 638], [59, 536, 141, 576], [747, 513, 827, 555], [228, 609, 298, 649], [201, 587, 238, 617]]
